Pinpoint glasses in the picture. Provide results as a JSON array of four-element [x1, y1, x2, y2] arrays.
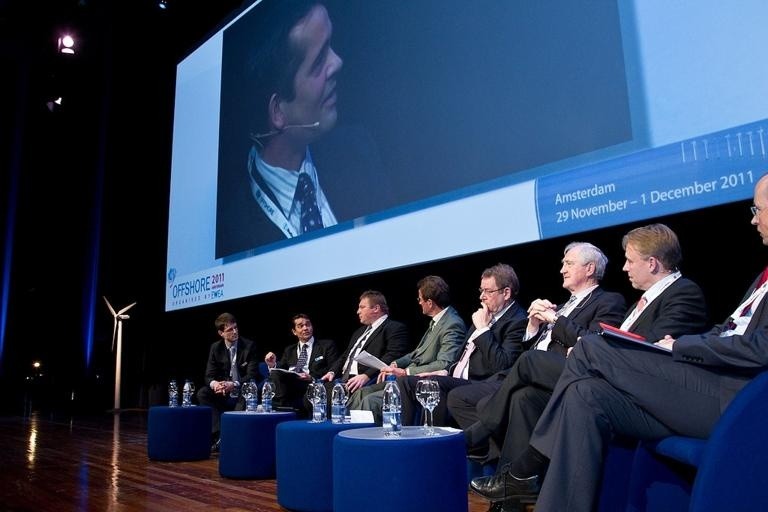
[[223, 324, 239, 333], [478, 287, 504, 295], [293, 323, 311, 328], [417, 297, 425, 301]]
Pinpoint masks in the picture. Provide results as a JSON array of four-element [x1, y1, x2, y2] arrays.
[[418, 300, 428, 314]]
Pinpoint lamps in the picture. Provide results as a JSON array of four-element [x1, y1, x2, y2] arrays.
[[56, 34, 76, 59]]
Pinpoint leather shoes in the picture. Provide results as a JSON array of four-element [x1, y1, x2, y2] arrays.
[[465, 443, 491, 466], [488, 501, 527, 512], [210, 443, 219, 453], [468, 461, 540, 504]]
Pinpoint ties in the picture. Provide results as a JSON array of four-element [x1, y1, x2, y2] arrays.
[[295, 172, 325, 234], [395, 320, 436, 369], [729, 265, 768, 328], [534, 296, 577, 348], [341, 325, 372, 383], [294, 344, 308, 373], [627, 298, 646, 312], [453, 315, 495, 378], [230, 343, 237, 377]]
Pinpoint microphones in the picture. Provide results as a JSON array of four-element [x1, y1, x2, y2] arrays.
[[245, 116, 320, 153]]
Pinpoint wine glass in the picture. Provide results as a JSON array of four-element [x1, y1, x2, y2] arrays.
[[421, 381, 439, 436], [188, 382, 194, 395], [307, 383, 320, 422], [339, 383, 348, 404], [416, 381, 430, 431], [269, 383, 276, 398], [241, 383, 251, 411], [173, 386, 177, 395]]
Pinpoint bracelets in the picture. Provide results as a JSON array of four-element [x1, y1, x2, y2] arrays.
[[234, 382, 238, 391], [327, 371, 334, 375], [552, 312, 560, 326]]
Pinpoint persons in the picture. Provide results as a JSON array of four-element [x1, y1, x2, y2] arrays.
[[227, 1, 343, 256], [446, 242, 627, 429], [463, 223, 707, 512], [258, 313, 337, 406], [399, 263, 529, 427], [343, 275, 468, 425], [198, 313, 257, 453], [469, 171, 768, 512], [320, 290, 408, 393]]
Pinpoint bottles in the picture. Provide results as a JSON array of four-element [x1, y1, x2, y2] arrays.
[[381, 373, 405, 438], [262, 377, 274, 413], [311, 377, 327, 421], [246, 378, 258, 411], [168, 379, 180, 407], [330, 379, 348, 424], [182, 379, 193, 406]]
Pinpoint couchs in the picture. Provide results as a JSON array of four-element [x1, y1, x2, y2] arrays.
[[622, 368, 768, 512]]
